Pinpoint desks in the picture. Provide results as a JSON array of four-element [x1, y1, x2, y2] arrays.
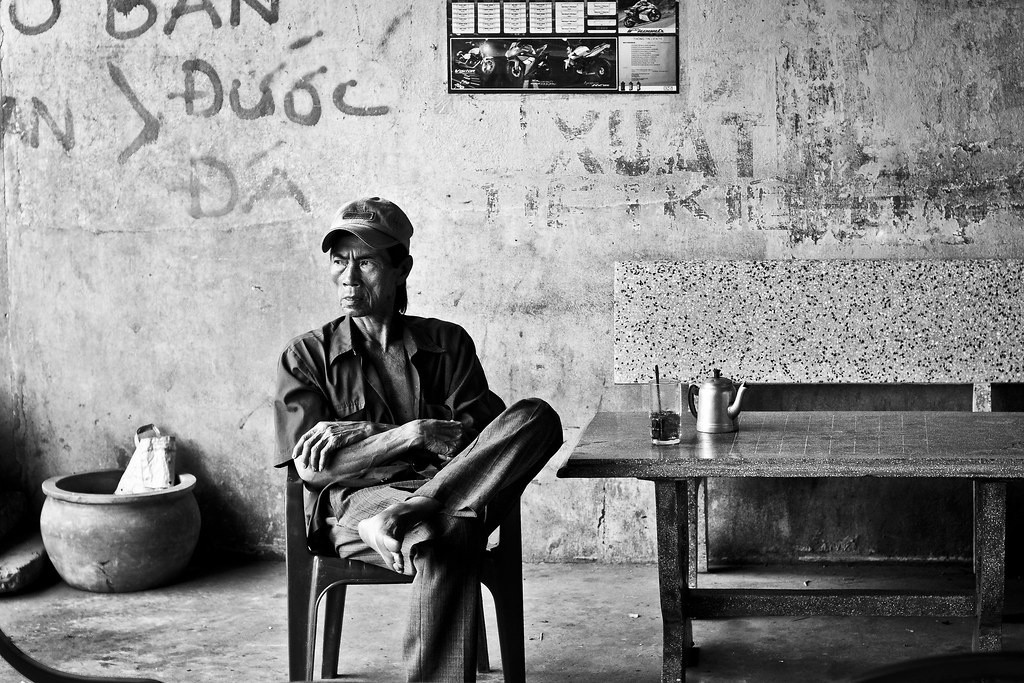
[[556, 405, 1024, 683]]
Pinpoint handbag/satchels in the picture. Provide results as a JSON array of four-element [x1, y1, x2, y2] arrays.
[[115, 424, 176, 493]]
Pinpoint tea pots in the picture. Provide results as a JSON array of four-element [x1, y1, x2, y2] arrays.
[[688, 368, 748, 434]]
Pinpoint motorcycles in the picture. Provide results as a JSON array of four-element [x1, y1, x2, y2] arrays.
[[559, 38, 616, 88], [502, 39, 554, 85], [622, 0, 663, 29], [451, 38, 500, 79]]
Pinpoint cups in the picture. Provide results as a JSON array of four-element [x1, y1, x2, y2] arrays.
[[648, 379, 682, 445]]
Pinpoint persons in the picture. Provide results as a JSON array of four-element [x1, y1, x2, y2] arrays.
[[274, 198, 563, 683]]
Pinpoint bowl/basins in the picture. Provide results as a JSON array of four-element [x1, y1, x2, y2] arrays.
[[41, 470, 202, 593]]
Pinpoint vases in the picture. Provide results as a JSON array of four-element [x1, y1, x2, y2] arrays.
[[38, 469, 199, 592]]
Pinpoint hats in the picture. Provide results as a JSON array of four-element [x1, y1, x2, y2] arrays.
[[321, 197, 413, 255]]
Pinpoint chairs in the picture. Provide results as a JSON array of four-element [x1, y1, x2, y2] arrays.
[[280, 462, 526, 683]]
